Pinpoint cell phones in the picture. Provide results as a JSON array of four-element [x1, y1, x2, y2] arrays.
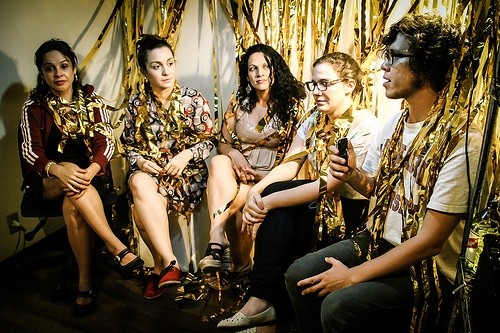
[[336, 138, 348, 175]]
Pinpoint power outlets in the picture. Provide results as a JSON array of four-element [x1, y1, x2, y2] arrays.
[[7, 212, 20, 235]]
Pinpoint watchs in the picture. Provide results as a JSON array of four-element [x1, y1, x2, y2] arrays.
[[129, 156, 142, 170]]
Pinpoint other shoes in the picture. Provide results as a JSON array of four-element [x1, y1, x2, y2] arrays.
[[73, 290, 97, 317], [113, 249, 144, 275], [235, 326, 256, 333], [157, 261, 185, 288], [216, 305, 277, 327], [143, 273, 168, 300]]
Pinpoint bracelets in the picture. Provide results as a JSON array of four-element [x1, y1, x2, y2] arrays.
[[45, 162, 56, 178]]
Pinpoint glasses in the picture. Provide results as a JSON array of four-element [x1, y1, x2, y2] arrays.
[[385, 49, 412, 66], [305, 78, 342, 91]]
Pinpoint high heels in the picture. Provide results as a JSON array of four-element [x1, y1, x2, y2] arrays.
[[200, 242, 232, 271], [204, 259, 253, 292]]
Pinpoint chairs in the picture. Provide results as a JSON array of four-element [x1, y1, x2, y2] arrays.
[[21, 125, 118, 288]]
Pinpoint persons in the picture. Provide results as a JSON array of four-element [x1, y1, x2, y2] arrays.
[[285, 16, 483, 332], [19, 39, 144, 316], [118, 34, 214, 299], [217, 52, 380, 333], [198, 43, 306, 292]]
